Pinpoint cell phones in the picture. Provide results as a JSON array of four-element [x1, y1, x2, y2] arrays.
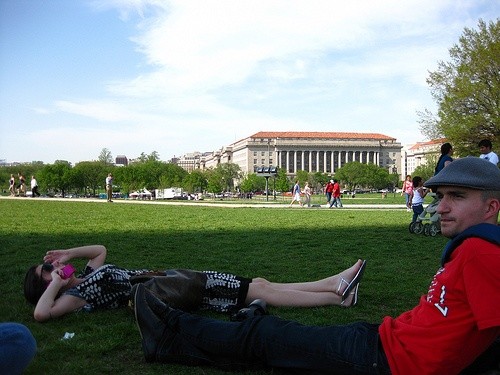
[[61, 263, 76, 279]]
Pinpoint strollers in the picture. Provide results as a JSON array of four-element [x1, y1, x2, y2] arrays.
[[409, 195, 442, 236]]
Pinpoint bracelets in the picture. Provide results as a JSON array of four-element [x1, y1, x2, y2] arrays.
[[409, 201, 411, 203]]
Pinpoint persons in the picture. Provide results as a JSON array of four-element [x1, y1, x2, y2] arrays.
[[106, 173, 113, 202], [401, 175, 414, 213], [188, 191, 204, 201], [131, 156, 500, 375], [132, 191, 155, 200], [434, 143, 455, 176], [325, 178, 343, 208], [23, 245, 366, 321], [289, 181, 302, 208], [303, 182, 311, 207], [31, 176, 40, 197], [0, 322, 37, 375], [478, 139, 499, 167], [408, 176, 430, 231], [9, 172, 26, 197], [237, 187, 253, 198]]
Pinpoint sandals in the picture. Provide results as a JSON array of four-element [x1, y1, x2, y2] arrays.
[[337, 259, 367, 300], [338, 282, 360, 307]]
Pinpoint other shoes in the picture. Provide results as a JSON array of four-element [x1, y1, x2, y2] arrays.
[[131, 285, 173, 321], [133, 283, 168, 363]]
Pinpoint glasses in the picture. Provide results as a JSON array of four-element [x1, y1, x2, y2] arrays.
[[40, 263, 54, 290]]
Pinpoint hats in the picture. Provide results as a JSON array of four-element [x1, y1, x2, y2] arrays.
[[330, 180, 334, 184], [423, 158, 500, 191]]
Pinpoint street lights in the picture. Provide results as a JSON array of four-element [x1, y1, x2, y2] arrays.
[[257, 166, 278, 202]]
